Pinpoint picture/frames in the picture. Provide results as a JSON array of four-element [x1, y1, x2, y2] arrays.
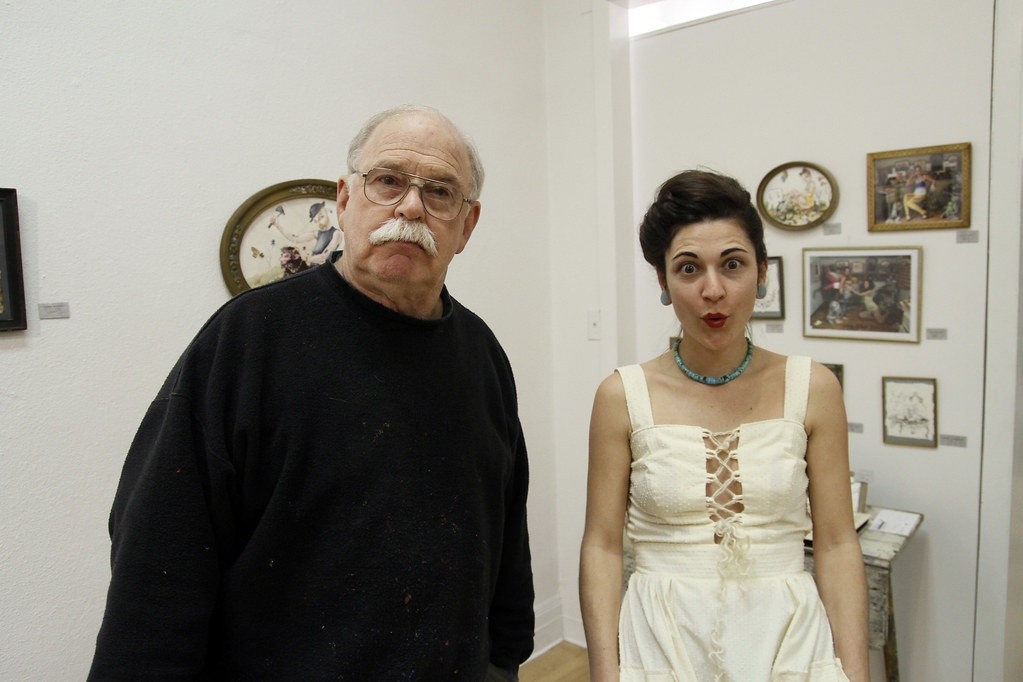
[[802, 245, 922, 344], [0, 187, 28, 333], [757, 161, 841, 233], [219, 178, 345, 300], [881, 376, 938, 448], [866, 142, 971, 231], [750, 255, 787, 319]]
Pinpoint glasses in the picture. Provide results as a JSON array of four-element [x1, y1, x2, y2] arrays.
[[347, 166, 477, 222]]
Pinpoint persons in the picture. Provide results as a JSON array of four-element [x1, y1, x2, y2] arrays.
[[577, 163, 870, 682], [903, 164, 936, 220], [85, 105, 535, 682], [849, 279, 893, 324]]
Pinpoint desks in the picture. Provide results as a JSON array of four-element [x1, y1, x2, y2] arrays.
[[803, 508, 923, 682]]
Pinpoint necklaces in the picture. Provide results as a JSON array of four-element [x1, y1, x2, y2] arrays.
[[674, 337, 753, 386]]
